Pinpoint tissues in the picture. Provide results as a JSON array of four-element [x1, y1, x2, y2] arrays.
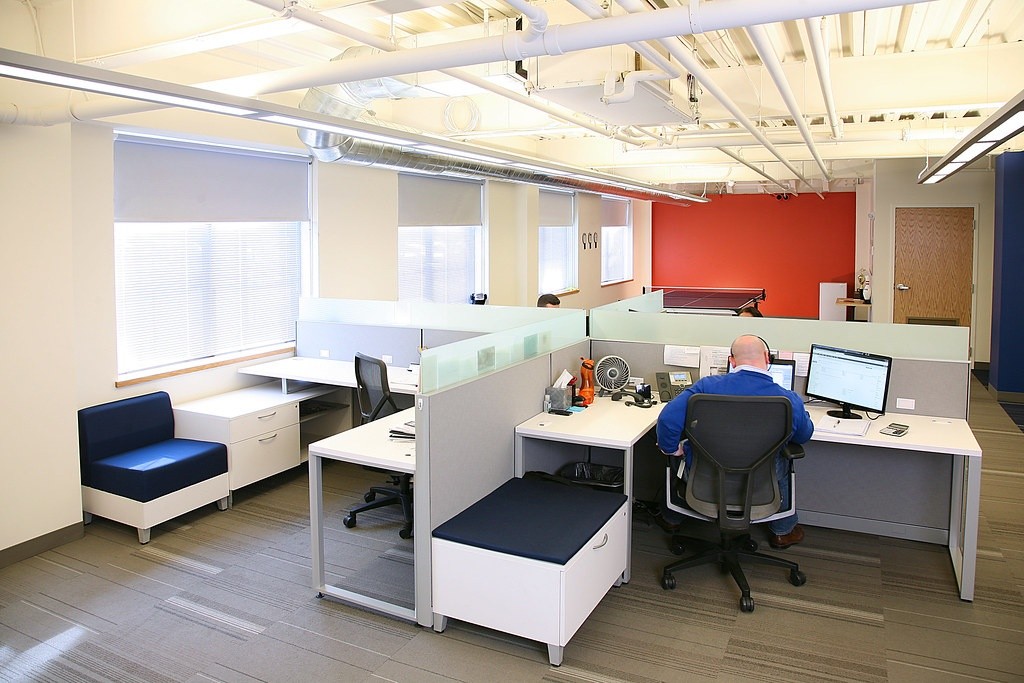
[[545, 368, 574, 412]]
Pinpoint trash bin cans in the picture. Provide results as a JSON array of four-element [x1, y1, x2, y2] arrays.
[[559, 462, 624, 494]]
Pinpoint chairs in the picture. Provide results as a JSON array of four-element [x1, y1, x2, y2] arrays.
[[78, 391, 229, 544], [343, 351, 413, 539], [666, 393, 805, 612]]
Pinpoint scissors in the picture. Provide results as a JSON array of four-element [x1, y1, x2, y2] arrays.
[[569, 376, 577, 385]]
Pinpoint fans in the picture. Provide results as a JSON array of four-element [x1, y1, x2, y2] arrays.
[[593, 354, 631, 397]]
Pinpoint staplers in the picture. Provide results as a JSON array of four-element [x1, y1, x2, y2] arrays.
[[572, 395, 588, 408]]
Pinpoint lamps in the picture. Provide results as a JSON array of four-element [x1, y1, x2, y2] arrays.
[[916, 20, 1024, 184], [0, 0, 712, 203]]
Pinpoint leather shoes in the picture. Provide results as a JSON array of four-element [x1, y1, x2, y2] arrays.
[[654, 510, 679, 534], [770, 526, 805, 549]]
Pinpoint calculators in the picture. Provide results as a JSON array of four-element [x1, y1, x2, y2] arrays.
[[878, 422, 910, 437]]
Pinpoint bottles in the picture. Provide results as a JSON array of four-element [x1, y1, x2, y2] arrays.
[[544, 395, 552, 413], [578, 357, 595, 404], [864, 276, 872, 304]]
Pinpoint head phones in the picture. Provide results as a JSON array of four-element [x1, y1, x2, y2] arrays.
[[730, 337, 774, 364]]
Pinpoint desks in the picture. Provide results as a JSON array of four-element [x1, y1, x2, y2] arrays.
[[835, 298, 872, 307], [239, 289, 981, 628], [642, 286, 766, 317]]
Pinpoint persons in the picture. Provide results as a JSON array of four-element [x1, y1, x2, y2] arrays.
[[537, 293, 560, 309], [656, 335, 807, 549]]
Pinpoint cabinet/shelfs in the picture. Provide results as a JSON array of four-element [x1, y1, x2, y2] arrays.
[[171, 380, 353, 510], [433, 477, 628, 667]]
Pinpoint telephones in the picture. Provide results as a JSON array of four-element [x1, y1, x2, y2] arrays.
[[611, 370, 694, 407]]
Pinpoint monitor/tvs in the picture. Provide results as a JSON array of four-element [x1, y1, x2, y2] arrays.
[[727, 356, 796, 391], [805, 344, 892, 420]]
